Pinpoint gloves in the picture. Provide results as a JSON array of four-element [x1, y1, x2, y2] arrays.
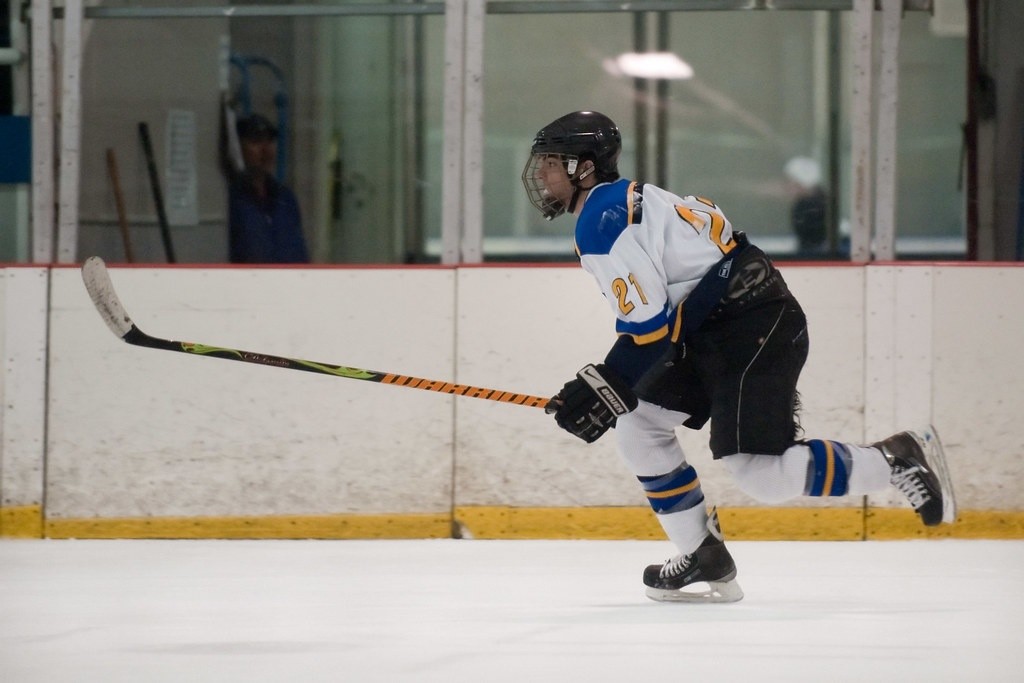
[[545, 363, 638, 443]]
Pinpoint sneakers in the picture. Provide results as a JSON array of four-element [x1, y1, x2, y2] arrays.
[[643, 507, 744, 603], [872, 424, 956, 526]]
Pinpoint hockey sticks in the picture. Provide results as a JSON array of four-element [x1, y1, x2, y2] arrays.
[[80, 257, 565, 411]]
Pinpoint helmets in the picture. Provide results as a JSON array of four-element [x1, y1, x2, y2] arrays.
[[531, 111, 622, 220]]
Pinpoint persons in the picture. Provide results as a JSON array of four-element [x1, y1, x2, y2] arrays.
[[521, 108, 957, 605], [228, 111, 307, 265]]
